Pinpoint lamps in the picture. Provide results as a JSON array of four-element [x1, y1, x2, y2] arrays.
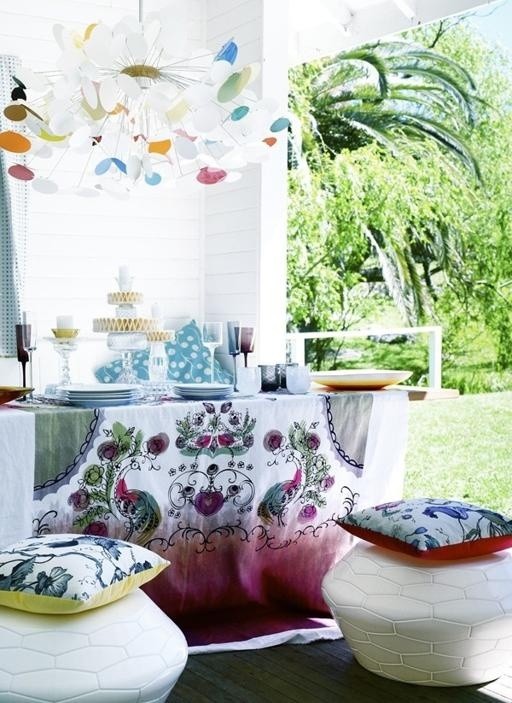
[[0, 1, 293, 198]]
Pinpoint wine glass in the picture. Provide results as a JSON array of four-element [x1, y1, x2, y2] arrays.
[[15, 324, 31, 401], [200, 321, 223, 382], [22, 311, 38, 402], [241, 326, 256, 368], [227, 320, 240, 392]]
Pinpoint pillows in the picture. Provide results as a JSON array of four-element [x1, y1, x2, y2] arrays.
[[333, 495, 511, 559], [0, 532, 172, 614]]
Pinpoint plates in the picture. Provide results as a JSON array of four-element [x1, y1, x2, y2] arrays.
[[1, 386, 35, 406], [66, 383, 138, 407], [174, 383, 234, 400], [310, 369, 413, 391]]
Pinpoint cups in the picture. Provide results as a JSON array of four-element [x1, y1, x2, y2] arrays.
[[236, 362, 310, 397]]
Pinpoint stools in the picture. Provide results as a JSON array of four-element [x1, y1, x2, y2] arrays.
[[317, 543, 511, 691], [2, 590, 189, 703]]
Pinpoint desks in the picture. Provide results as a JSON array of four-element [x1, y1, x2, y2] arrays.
[[0, 389, 407, 655]]
